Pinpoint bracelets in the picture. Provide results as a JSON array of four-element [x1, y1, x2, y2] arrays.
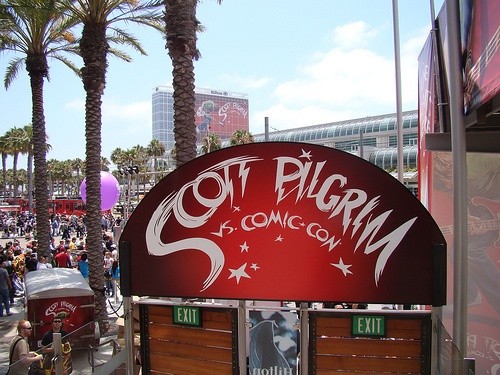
[[9, 285, 12, 286]]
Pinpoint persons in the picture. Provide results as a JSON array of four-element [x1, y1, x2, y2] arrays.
[[322, 302, 368, 309], [0, 213, 130, 306], [41, 316, 71, 375], [6, 320, 42, 375], [0, 260, 12, 317]]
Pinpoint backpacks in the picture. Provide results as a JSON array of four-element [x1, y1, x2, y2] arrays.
[[61, 342, 72, 375]]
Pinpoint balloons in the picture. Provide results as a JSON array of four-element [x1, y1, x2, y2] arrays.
[[81, 172, 119, 209]]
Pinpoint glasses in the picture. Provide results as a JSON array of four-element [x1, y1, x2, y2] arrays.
[[53, 321, 61, 323], [25, 327, 32, 330]]
